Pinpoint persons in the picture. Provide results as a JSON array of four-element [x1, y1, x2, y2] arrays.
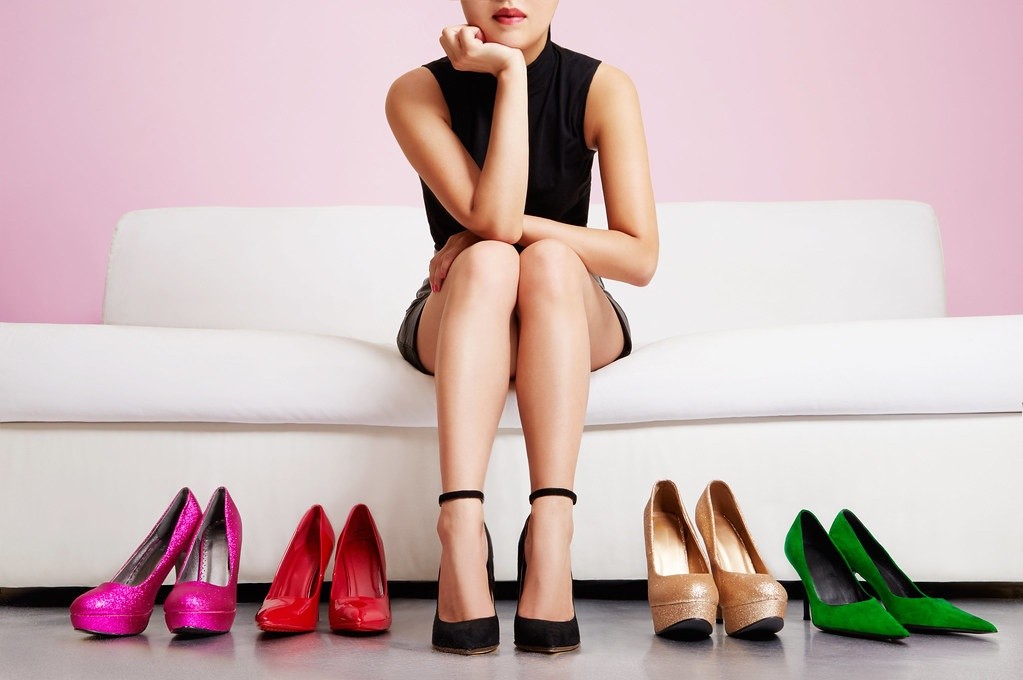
[[387, 0, 658, 655]]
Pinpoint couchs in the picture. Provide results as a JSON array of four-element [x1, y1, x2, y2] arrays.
[[0, 198, 1023, 583]]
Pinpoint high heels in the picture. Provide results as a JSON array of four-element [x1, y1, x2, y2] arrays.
[[784, 506, 998, 640], [70, 486, 243, 638], [643, 478, 719, 638], [694, 478, 787, 638], [254, 503, 335, 634], [327, 503, 393, 634], [511, 487, 581, 654], [433, 491, 498, 655]]
